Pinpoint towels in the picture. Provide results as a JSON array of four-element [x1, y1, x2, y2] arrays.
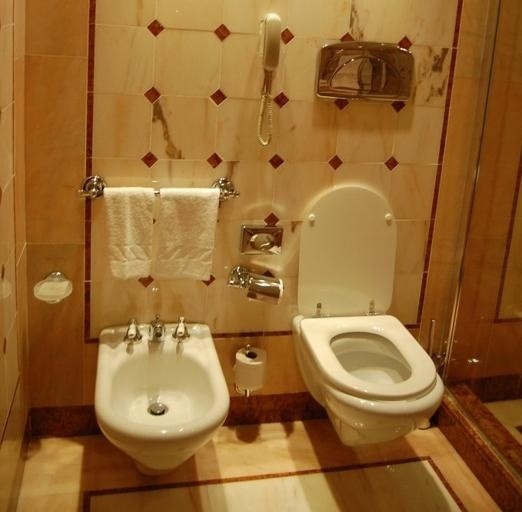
[[153, 186, 220, 282], [103, 186, 155, 281]]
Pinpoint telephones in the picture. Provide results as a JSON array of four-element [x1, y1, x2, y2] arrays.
[[256, 13, 282, 145]]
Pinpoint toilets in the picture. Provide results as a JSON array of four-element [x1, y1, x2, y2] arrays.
[[292, 183, 446, 448], [95, 320, 229, 474]]
